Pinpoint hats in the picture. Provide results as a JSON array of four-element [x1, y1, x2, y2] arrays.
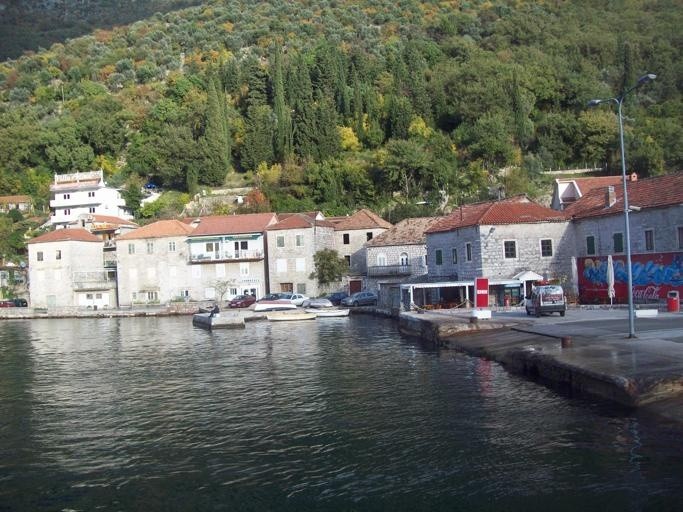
[[228, 294, 256, 308], [0, 298, 28, 307], [525, 284, 568, 316], [261, 292, 377, 306]]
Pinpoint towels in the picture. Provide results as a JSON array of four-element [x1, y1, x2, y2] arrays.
[[667, 290, 680, 312]]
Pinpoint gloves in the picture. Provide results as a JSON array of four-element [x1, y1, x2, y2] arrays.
[[266, 308, 352, 321], [249, 300, 296, 311]]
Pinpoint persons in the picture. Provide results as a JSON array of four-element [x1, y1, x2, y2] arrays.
[[207, 302, 220, 317]]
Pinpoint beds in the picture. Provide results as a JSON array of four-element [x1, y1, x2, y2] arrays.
[[569, 255, 579, 304], [605, 254, 616, 306]]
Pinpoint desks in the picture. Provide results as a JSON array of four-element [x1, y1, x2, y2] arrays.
[[585, 74, 657, 339]]
